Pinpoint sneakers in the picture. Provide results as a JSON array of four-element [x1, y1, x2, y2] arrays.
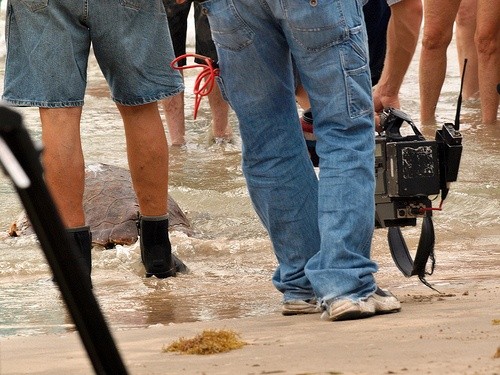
[[281, 296, 326, 316], [326, 285, 402, 322]]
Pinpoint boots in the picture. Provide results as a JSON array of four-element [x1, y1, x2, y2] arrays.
[[140, 212, 180, 279], [52, 224, 93, 288]]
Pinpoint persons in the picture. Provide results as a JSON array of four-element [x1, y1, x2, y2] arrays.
[[198, 0, 423, 321], [362, 0, 500, 138], [161, 0, 243, 147], [2, 1, 197, 289]]
